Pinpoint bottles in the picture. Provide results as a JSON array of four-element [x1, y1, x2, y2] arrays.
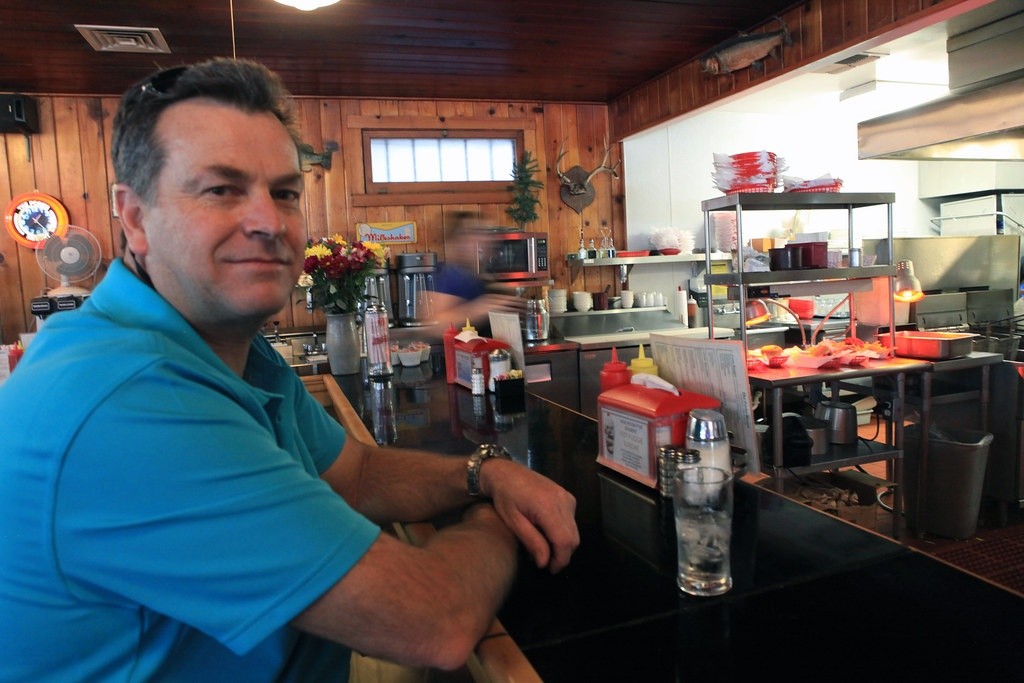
[[660, 411, 732, 505], [461, 318, 478, 334], [469, 367, 485, 396], [473, 396, 514, 431], [369, 376, 397, 445], [487, 349, 510, 392], [577, 238, 616, 258], [443, 322, 461, 383], [364, 306, 394, 376]]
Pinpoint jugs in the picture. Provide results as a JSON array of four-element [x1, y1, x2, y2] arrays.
[[523, 296, 549, 341]]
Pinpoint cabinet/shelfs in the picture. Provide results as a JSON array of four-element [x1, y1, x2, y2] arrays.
[[700, 192, 905, 541]]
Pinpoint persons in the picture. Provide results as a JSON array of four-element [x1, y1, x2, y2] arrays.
[[0, 57, 582, 683], [413, 207, 529, 344]]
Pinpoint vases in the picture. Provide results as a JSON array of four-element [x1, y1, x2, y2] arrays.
[[327, 304, 363, 380]]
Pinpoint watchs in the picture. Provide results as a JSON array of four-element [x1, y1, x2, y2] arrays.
[[465, 443, 512, 502]]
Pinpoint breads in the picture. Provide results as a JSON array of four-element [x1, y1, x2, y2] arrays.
[[760, 345, 783, 357], [809, 344, 832, 357]]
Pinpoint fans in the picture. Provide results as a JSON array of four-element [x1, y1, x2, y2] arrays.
[[30, 224, 106, 296]]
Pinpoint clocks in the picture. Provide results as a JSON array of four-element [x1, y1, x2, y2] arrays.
[[7, 192, 72, 258]]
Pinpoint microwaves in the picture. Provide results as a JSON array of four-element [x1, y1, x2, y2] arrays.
[[459, 231, 549, 281]]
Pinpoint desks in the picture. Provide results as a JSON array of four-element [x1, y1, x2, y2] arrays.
[[300, 356, 1024, 683]]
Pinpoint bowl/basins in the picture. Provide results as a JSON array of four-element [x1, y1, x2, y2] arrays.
[[789, 299, 815, 319], [796, 416, 830, 455], [391, 352, 400, 366], [815, 401, 857, 443], [548, 288, 663, 314], [397, 350, 422, 367], [420, 346, 433, 361]]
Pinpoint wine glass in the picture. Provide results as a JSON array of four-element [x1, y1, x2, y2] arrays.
[[815, 298, 849, 318]]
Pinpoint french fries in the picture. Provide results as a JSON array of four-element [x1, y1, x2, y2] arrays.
[[864, 340, 887, 350]]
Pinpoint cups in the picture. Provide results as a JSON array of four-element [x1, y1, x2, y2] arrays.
[[672, 465, 733, 597], [768, 248, 790, 270], [790, 246, 803, 269], [848, 248, 861, 268]]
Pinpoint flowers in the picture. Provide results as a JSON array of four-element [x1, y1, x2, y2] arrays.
[[296, 231, 384, 318]]
